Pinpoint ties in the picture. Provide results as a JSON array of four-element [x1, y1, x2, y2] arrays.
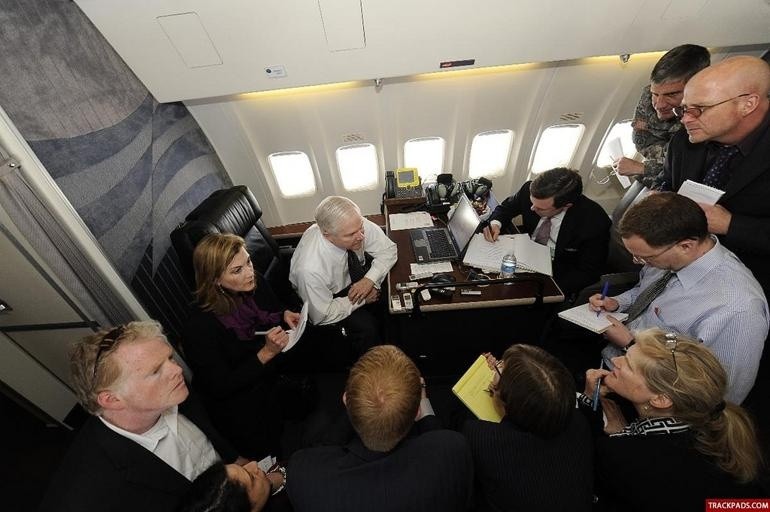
[[535, 217, 551, 245], [347, 249, 363, 282], [622, 271, 674, 324], [702, 147, 738, 186]]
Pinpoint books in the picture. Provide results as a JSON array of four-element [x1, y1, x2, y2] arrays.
[[557, 300, 629, 335], [462, 233, 556, 276]]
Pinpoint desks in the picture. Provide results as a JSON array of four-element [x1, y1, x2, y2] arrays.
[[381, 191, 567, 316]]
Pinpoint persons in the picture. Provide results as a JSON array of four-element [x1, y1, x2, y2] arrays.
[[612, 43, 712, 189], [589, 191, 770, 408], [179, 232, 320, 454], [664, 56, 770, 298], [183, 461, 287, 512], [460, 344, 603, 512], [284, 346, 474, 512], [39, 318, 236, 512], [482, 167, 613, 289], [290, 196, 394, 337], [576, 330, 764, 512]]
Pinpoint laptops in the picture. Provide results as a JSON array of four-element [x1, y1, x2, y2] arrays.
[[409, 192, 482, 264]]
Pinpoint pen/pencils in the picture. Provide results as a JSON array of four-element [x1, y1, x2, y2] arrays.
[[483, 388, 498, 393], [487, 219, 496, 243], [593, 358, 606, 412], [596, 281, 610, 318], [253, 331, 289, 336]]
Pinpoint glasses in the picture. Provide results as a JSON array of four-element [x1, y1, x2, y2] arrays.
[[633, 236, 696, 265], [665, 332, 679, 371], [492, 360, 503, 379], [92, 327, 124, 378], [592, 157, 619, 184], [673, 93, 750, 117]]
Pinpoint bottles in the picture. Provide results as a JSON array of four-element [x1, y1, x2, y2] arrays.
[[500, 250, 518, 287]]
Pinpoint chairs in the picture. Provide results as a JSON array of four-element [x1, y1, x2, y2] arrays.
[[558, 178, 658, 341], [168, 183, 305, 314]]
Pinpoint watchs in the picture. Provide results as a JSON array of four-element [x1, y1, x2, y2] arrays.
[[621, 339, 636, 354]]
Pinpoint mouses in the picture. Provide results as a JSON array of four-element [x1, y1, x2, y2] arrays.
[[432, 274, 450, 282]]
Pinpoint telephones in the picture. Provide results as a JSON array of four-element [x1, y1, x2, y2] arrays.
[[384, 168, 422, 199]]
